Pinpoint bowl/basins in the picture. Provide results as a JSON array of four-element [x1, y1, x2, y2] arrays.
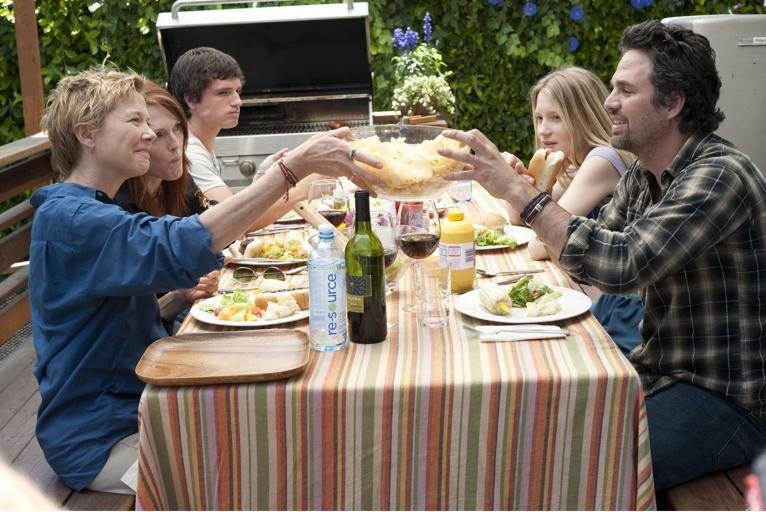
[[346, 123, 472, 203]]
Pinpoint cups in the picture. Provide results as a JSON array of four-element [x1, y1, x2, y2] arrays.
[[414, 262, 452, 329], [448, 179, 473, 203]]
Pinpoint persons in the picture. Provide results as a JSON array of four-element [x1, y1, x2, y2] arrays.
[[500, 66, 648, 363], [170, 47, 338, 234], [28, 67, 386, 496], [437, 20, 766, 492], [111, 80, 290, 335]]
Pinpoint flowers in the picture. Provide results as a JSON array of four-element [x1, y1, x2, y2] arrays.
[[389, 10, 458, 116]]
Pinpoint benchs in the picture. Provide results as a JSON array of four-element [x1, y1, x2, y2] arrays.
[[662, 452, 766, 510], [1, 129, 133, 512]]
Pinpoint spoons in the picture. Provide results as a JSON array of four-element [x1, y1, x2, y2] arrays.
[[476, 269, 546, 278]]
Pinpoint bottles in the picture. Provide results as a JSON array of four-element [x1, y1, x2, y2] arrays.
[[438, 210, 476, 294], [345, 190, 387, 344], [308, 223, 348, 353]]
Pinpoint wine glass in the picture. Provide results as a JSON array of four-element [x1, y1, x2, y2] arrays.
[[396, 199, 441, 312], [372, 226, 398, 267], [307, 179, 348, 228]]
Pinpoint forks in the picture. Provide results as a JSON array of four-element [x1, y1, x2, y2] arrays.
[[461, 324, 571, 337]]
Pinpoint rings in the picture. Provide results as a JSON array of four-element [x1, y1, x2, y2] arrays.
[[350, 172, 357, 182], [349, 148, 355, 161]]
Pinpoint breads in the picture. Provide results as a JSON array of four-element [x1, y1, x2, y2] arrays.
[[255, 290, 309, 309]]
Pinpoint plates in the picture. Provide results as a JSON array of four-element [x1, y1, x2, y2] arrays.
[[475, 225, 535, 251], [452, 287, 593, 324], [187, 217, 310, 328]]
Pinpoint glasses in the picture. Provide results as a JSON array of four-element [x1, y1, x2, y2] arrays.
[[233, 266, 286, 285]]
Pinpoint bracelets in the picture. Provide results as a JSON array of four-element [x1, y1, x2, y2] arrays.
[[519, 192, 553, 229], [277, 159, 299, 187]]
